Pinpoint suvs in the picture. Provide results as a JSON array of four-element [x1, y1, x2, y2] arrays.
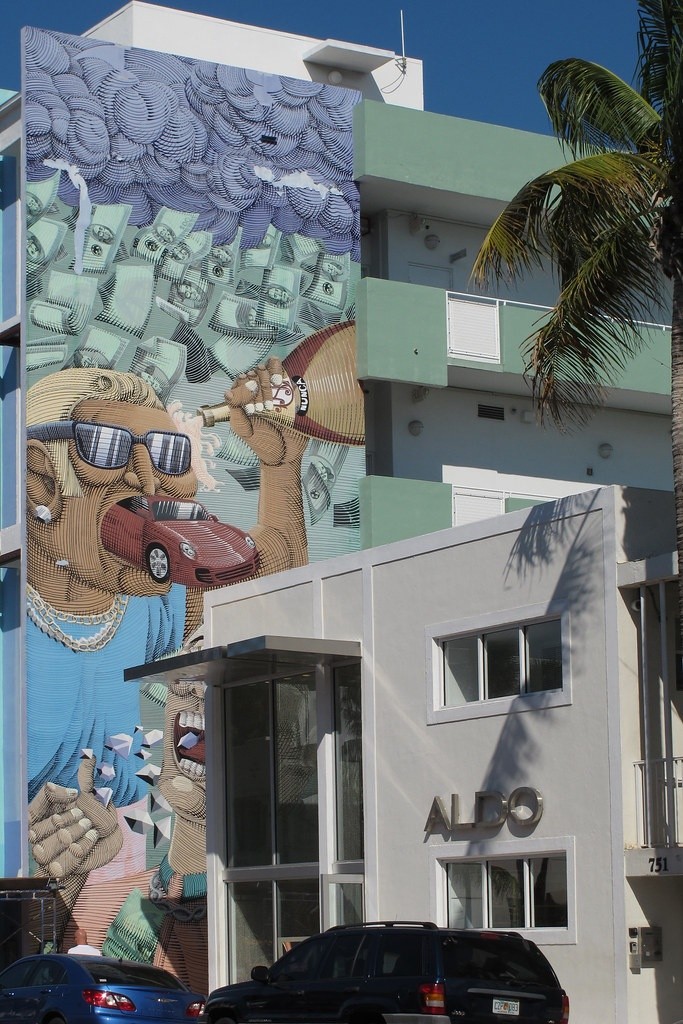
[[203, 920, 569, 1024]]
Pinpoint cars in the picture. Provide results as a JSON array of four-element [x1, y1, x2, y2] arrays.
[[0, 953, 208, 1024]]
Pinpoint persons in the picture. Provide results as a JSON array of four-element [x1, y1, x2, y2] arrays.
[[67, 929, 102, 957]]
